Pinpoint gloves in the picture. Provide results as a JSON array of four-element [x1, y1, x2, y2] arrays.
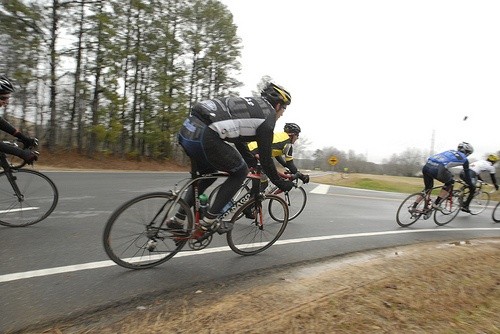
[[22, 137, 39, 148], [300, 174, 310, 185], [20, 150, 39, 166], [277, 177, 292, 192], [244, 154, 258, 168]]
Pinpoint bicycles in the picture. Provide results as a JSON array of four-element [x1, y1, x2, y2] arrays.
[[101, 147, 292, 271], [208, 159, 309, 223], [0, 140, 59, 228], [451, 177, 491, 215], [395, 176, 470, 228], [492, 201, 500, 222]]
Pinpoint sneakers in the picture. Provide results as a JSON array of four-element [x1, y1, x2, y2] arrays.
[[166, 217, 185, 244], [198, 220, 233, 234]]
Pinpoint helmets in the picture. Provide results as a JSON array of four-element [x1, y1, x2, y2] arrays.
[[458, 142, 473, 153], [269, 81, 291, 106], [283, 123, 301, 135], [488, 154, 499, 162], [0, 75, 14, 94]]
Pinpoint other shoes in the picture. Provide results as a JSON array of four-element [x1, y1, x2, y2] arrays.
[[409, 207, 417, 212], [432, 204, 443, 210], [460, 209, 471, 212]]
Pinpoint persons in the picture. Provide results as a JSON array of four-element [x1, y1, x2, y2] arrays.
[[0, 75, 38, 165], [459, 154, 500, 213], [166, 82, 293, 248], [242, 123, 309, 219], [412, 142, 473, 212]]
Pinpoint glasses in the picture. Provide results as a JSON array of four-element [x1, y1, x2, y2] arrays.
[[0, 96, 9, 100]]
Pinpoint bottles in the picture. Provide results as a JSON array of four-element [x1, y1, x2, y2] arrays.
[[219, 199, 235, 214], [263, 185, 273, 196], [199, 194, 208, 219]]
